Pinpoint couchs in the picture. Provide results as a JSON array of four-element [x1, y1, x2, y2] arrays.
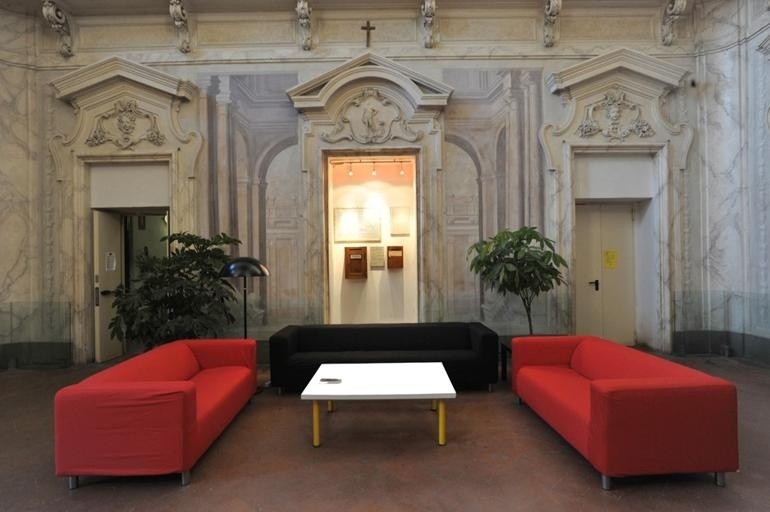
[[509, 334, 740, 490], [53, 337, 257, 491], [268, 322, 500, 396]]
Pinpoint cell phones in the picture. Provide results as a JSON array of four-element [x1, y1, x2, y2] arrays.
[[321, 378, 341, 383]]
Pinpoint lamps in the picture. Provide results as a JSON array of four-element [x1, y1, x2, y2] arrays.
[[219, 256, 271, 338]]
